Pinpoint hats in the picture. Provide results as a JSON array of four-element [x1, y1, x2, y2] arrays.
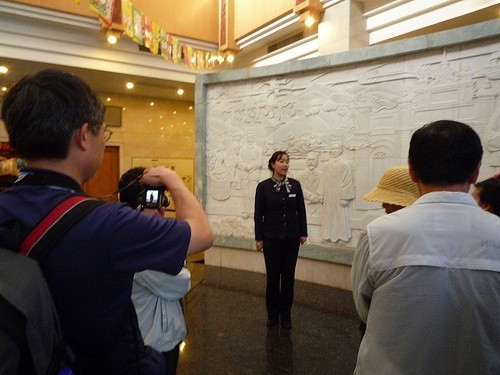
[[361, 166, 421, 208]]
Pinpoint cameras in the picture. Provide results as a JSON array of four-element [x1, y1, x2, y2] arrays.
[[135, 184, 170, 210]]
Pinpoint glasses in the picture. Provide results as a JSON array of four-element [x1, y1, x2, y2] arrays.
[[89, 123, 113, 143]]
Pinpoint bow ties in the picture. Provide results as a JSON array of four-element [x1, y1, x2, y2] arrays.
[[270, 176, 293, 194]]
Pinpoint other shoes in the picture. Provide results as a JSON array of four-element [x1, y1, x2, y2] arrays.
[[282, 319, 293, 330], [267, 320, 279, 328]]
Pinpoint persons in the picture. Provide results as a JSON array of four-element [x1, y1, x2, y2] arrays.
[[0, 69, 214, 375], [472, 174, 500, 217], [298, 144, 355, 244], [212, 132, 263, 219], [150, 192, 154, 202], [362, 166, 420, 214], [349, 120, 500, 375], [254, 150, 308, 330]]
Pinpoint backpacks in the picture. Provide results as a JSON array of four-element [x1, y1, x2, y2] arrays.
[[0, 194, 110, 375]]
